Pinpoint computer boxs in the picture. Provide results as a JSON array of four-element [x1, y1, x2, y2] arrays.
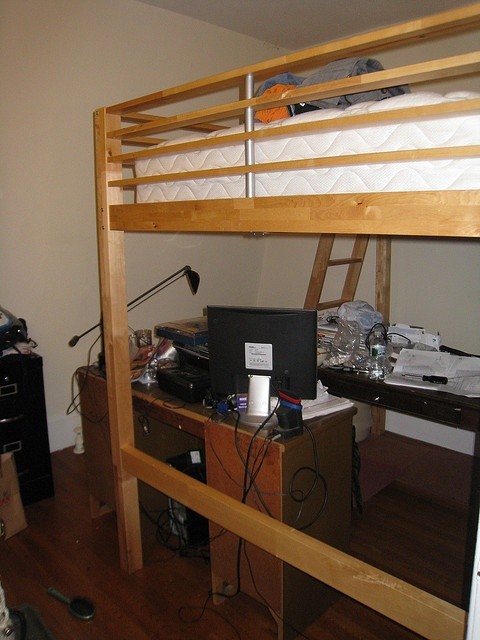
[[165, 449, 206, 542]]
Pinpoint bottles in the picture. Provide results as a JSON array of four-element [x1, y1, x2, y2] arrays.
[[369, 327, 387, 379]]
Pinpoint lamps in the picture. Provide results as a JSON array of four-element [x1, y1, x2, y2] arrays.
[[67, 265, 206, 344]]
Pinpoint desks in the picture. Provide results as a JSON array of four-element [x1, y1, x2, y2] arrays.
[[317, 305, 480, 434], [1, 353, 54, 504], [78, 353, 360, 637]]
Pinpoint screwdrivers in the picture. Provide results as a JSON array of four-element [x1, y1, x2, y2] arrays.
[[401, 373, 448, 385]]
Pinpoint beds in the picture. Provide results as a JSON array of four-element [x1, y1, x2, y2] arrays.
[[96, 3, 480, 640]]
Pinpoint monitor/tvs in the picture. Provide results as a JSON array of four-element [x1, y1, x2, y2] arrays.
[[207, 305, 317, 398]]
